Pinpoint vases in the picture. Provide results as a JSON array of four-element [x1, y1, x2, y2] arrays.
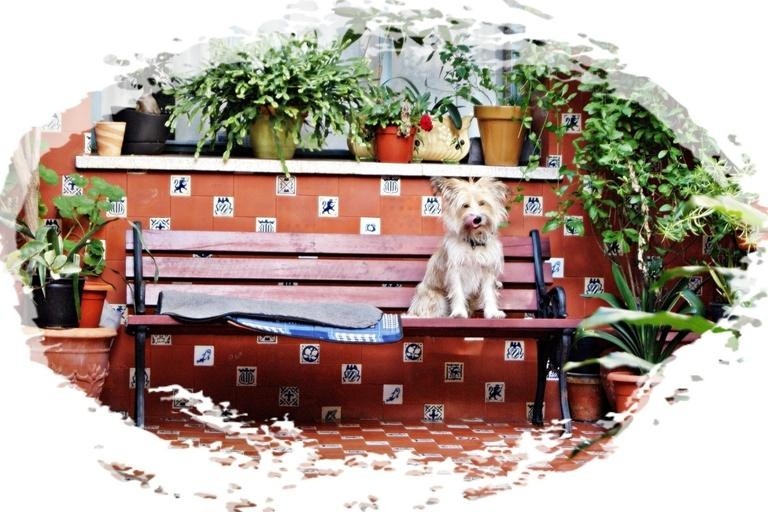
[[116, 85, 167, 154], [78, 281, 113, 329], [17, 325, 118, 402]]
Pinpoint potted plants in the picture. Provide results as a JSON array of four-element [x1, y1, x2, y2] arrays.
[[566, 261, 741, 426], [0, 167, 120, 330]]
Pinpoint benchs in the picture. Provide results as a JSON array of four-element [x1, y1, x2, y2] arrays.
[[126, 219, 572, 439]]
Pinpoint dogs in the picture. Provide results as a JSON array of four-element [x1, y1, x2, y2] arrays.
[[402, 170, 510, 321]]
